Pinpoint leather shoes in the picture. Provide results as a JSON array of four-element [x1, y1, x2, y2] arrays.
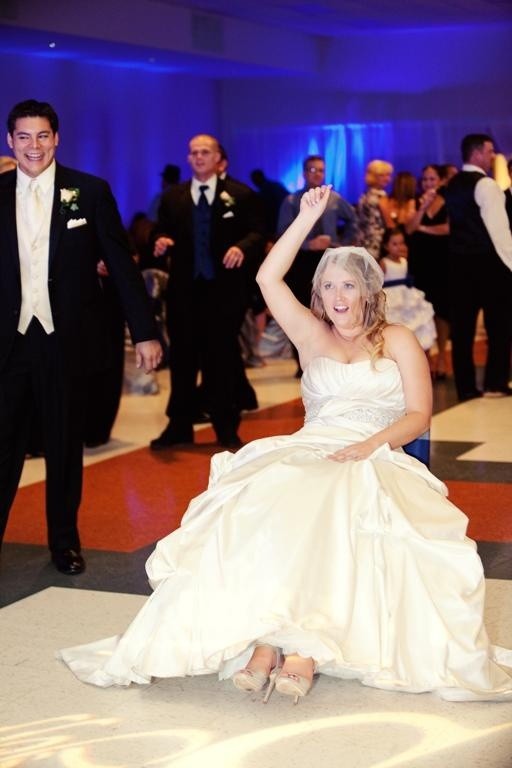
[[217, 429, 240, 448], [52, 550, 85, 575], [151, 425, 192, 447]]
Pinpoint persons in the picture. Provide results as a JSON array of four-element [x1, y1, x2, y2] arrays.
[[0, 99, 162, 576], [97, 132, 512, 453], [61, 185, 511, 706]]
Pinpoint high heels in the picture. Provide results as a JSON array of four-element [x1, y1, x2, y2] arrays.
[[234, 648, 281, 704], [276, 654, 314, 705]]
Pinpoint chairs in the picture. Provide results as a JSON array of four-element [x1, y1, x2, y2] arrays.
[[401, 428, 430, 472]]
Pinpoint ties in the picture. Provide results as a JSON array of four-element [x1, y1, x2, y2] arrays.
[[195, 186, 214, 280], [27, 180, 42, 244]]
